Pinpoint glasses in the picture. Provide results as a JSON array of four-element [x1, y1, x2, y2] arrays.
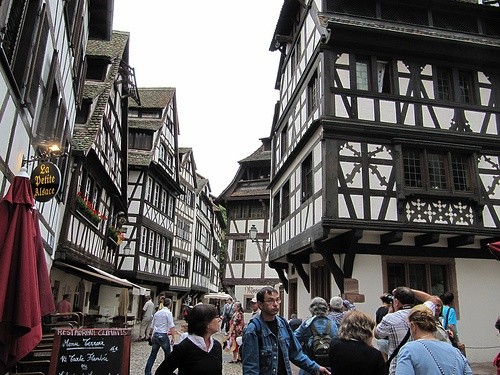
[[215, 315, 222, 319], [264, 297, 281, 303]]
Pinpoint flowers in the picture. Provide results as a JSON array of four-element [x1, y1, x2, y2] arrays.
[[77, 192, 107, 223], [109, 221, 125, 245]]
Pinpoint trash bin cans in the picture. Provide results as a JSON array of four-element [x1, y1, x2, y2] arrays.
[[114, 315, 135, 329]]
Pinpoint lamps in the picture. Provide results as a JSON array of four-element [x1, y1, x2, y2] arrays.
[[123, 244, 131, 256], [20, 137, 61, 167], [249, 225, 271, 243]]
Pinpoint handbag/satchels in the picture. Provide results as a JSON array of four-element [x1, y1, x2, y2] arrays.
[[221, 318, 225, 329], [225, 313, 229, 318]]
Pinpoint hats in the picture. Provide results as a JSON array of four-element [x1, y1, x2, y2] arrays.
[[250, 297, 257, 302], [221, 336, 236, 352]]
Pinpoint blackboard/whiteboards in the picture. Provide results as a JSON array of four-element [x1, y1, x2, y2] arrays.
[[49, 329, 131, 375]]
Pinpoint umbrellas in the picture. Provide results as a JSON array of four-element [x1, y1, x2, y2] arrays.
[[0, 163, 57, 375]]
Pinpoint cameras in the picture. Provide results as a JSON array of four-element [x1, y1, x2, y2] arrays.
[[379, 292, 394, 304]]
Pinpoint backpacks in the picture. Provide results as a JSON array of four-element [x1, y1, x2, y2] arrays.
[[307, 317, 332, 371]]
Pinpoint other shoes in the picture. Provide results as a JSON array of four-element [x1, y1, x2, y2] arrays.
[[237, 359, 242, 363], [228, 359, 239, 364], [134, 338, 143, 342], [142, 336, 150, 341], [224, 331, 229, 335]]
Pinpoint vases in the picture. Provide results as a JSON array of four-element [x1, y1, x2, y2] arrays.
[[109, 234, 118, 243], [77, 202, 99, 227]]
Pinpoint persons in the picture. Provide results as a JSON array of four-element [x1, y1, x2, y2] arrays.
[[493, 319, 500, 375], [133, 287, 473, 375], [395, 304, 473, 375], [57, 294, 72, 322], [154, 304, 222, 375], [241, 287, 331, 375]]
